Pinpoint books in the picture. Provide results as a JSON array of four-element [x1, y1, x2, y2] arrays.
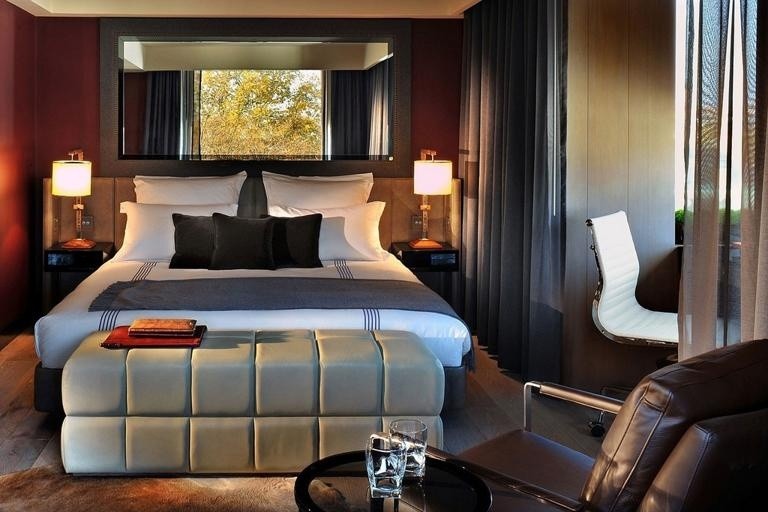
[[100, 318, 207, 349]]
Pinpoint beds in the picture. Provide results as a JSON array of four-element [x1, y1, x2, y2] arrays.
[[33, 178, 472, 413]]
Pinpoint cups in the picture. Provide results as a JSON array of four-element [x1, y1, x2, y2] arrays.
[[389, 419, 429, 478], [365, 432, 408, 499]]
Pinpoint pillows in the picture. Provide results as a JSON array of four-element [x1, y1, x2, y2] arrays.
[[112, 170, 387, 270]]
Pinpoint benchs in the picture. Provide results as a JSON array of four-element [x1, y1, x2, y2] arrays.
[[61, 330, 446, 474]]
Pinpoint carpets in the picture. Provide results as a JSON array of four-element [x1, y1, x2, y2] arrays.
[[0, 464, 349, 512]]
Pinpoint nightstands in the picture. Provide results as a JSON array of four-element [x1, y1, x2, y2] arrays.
[[44, 242, 113, 307], [391, 242, 459, 300]]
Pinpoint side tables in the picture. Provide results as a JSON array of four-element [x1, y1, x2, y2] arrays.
[[294, 450, 493, 512]]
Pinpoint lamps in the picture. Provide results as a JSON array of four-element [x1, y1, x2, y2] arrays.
[[408, 148, 453, 249], [51, 148, 96, 248]]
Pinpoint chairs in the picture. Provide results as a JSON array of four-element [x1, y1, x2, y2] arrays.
[[585, 210, 740, 437], [367, 338, 767, 512]]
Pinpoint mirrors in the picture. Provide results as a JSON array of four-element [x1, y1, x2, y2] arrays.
[[117, 35, 395, 161]]
